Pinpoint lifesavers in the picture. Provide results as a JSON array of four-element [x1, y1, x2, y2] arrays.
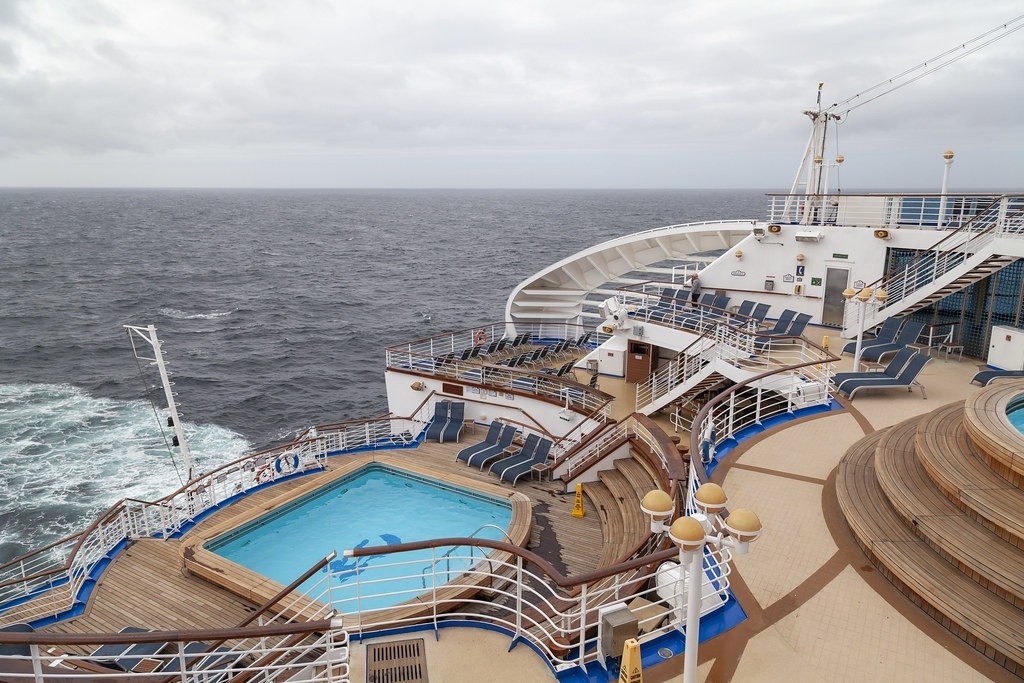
[[475, 331, 486, 345], [256, 464, 274, 483], [275, 452, 299, 475]]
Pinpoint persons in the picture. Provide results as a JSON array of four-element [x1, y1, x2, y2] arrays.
[[690, 272, 701, 312]]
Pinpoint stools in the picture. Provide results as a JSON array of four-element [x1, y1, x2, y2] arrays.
[[669, 435, 691, 464], [549, 636, 571, 661]]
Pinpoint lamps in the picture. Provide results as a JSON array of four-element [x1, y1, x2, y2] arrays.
[[753, 227, 766, 242], [735, 249, 743, 259], [795, 231, 826, 242], [796, 253, 805, 262]]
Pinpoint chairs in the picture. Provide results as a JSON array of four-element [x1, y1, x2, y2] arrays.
[[969, 370, 1024, 386], [424, 401, 466, 444], [455, 421, 553, 488], [399, 330, 599, 399], [635, 288, 814, 353], [829, 317, 932, 401], [47, 626, 246, 683]]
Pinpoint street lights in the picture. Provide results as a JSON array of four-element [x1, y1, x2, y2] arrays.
[[841, 287, 890, 372], [639, 482, 762, 683], [814, 153, 846, 226], [937, 150, 956, 230]]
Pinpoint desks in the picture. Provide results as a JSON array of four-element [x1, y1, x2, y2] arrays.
[[937, 342, 965, 362], [130, 658, 164, 673], [531, 463, 551, 485], [626, 597, 670, 634], [462, 419, 475, 434], [503, 446, 521, 458], [586, 359, 598, 375], [859, 361, 887, 374], [905, 343, 931, 357], [722, 397, 752, 421]]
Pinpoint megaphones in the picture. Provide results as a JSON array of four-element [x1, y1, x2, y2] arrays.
[[874, 230, 889, 238], [768, 225, 782, 233]]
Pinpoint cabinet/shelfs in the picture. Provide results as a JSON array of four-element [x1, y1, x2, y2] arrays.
[[986, 325, 1024, 371], [624, 339, 659, 387]]
